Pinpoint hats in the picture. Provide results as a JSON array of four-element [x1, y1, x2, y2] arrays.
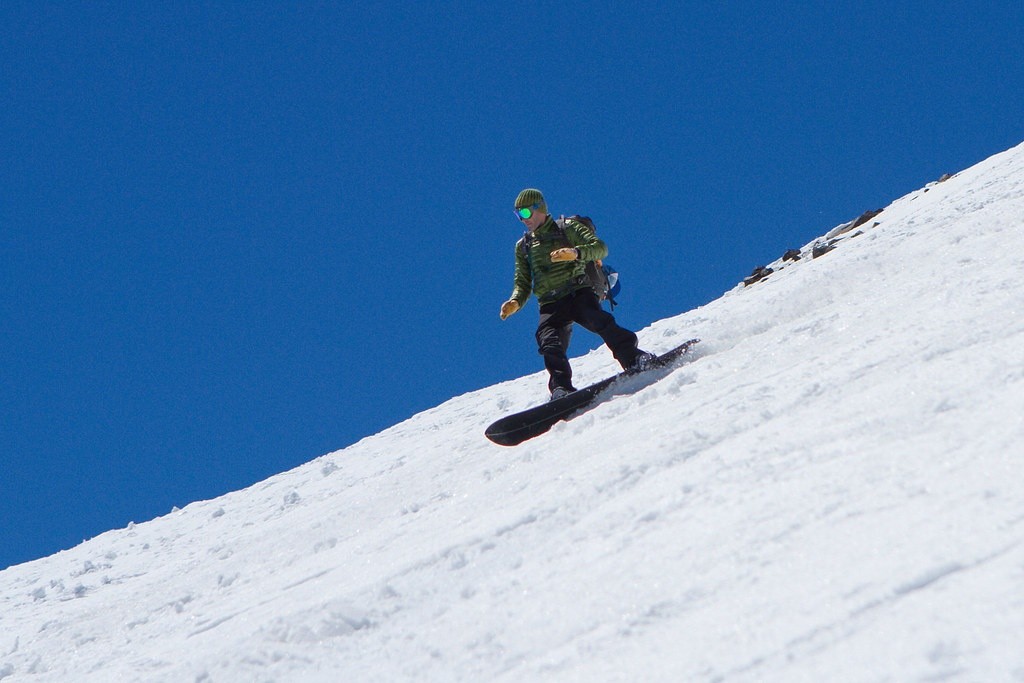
[[515, 189, 546, 213]]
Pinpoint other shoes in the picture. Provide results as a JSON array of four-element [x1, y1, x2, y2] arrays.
[[551, 387, 576, 402], [623, 354, 658, 370]]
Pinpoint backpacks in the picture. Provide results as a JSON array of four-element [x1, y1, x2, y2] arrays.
[[523, 215, 621, 300]]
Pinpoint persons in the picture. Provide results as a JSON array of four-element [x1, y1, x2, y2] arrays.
[[499, 188, 659, 402]]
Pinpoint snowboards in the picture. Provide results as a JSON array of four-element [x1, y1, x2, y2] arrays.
[[485, 338, 702, 446]]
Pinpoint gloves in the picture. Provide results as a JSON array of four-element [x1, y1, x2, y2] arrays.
[[500, 300, 519, 320], [550, 248, 578, 263]]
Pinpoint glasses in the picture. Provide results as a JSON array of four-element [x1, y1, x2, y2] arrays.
[[513, 201, 543, 222]]
